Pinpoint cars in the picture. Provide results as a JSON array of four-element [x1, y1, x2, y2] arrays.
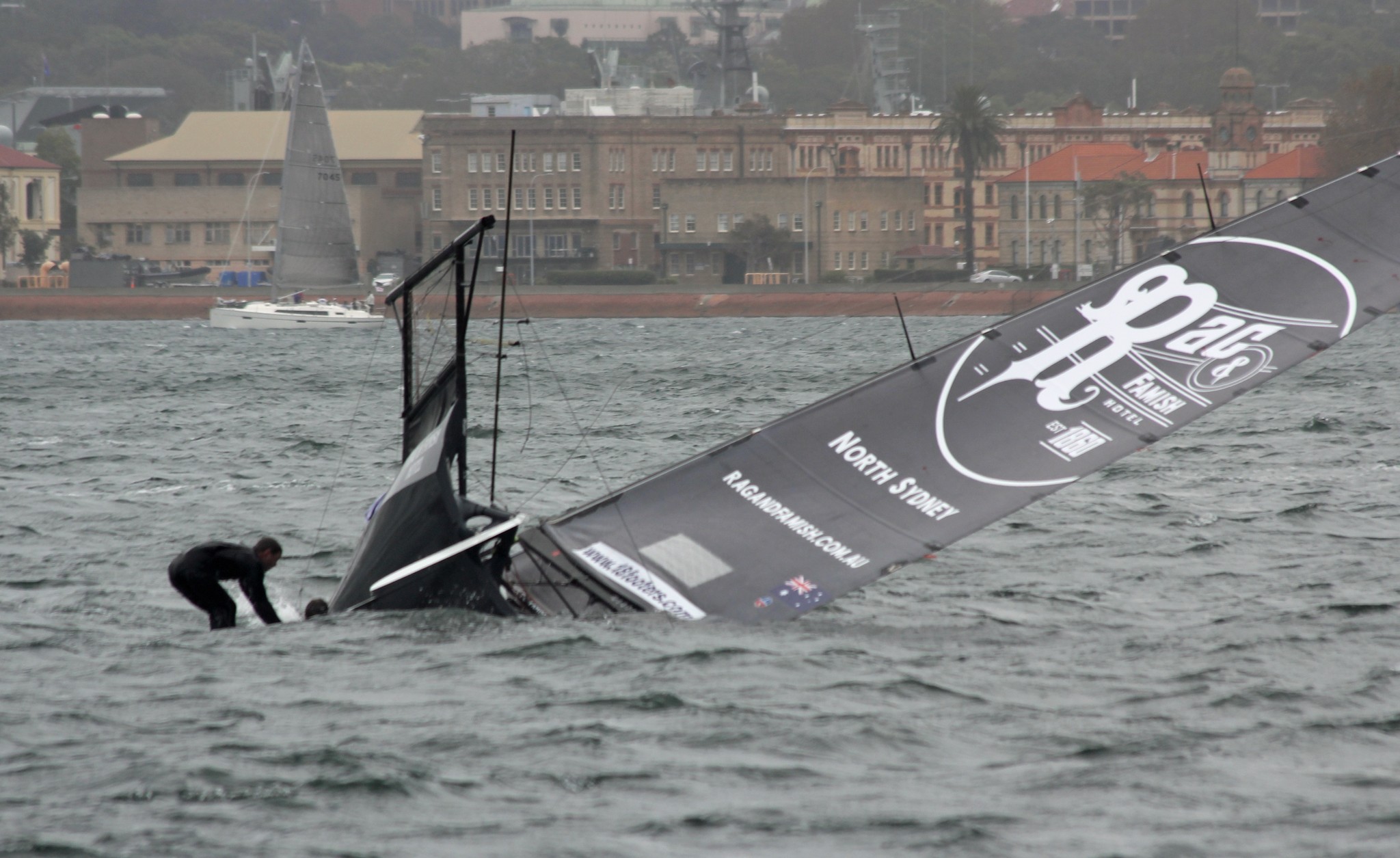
[[971, 268, 1020, 285], [372, 273, 398, 289]]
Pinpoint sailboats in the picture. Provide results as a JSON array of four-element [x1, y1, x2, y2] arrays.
[[209, 38, 385, 329], [306, 148, 1399, 625]]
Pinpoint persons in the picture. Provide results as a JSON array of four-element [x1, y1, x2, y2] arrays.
[[167, 537, 285, 632]]
